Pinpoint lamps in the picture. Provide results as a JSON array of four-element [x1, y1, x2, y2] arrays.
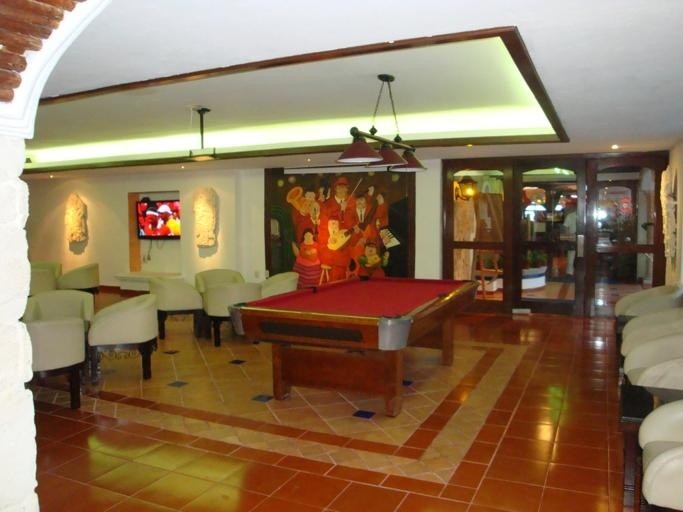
[[189, 108, 217, 161], [523, 202, 547, 211], [455, 174, 478, 200], [336, 75, 427, 173]]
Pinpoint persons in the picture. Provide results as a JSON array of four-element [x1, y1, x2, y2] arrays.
[[284, 174, 392, 289], [135, 196, 180, 238]]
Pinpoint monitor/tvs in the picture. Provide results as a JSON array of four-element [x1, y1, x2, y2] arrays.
[[135, 200, 182, 240]]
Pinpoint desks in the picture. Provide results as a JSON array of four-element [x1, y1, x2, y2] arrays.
[[228, 276, 483, 412]]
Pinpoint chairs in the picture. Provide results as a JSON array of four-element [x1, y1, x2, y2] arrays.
[[613, 285, 683, 512], [203, 282, 262, 348], [195, 268, 245, 296], [87, 294, 159, 387], [149, 275, 212, 340], [55, 262, 100, 296], [30, 262, 62, 294], [24, 317, 85, 408], [24, 290, 94, 379], [261, 271, 299, 301]]
